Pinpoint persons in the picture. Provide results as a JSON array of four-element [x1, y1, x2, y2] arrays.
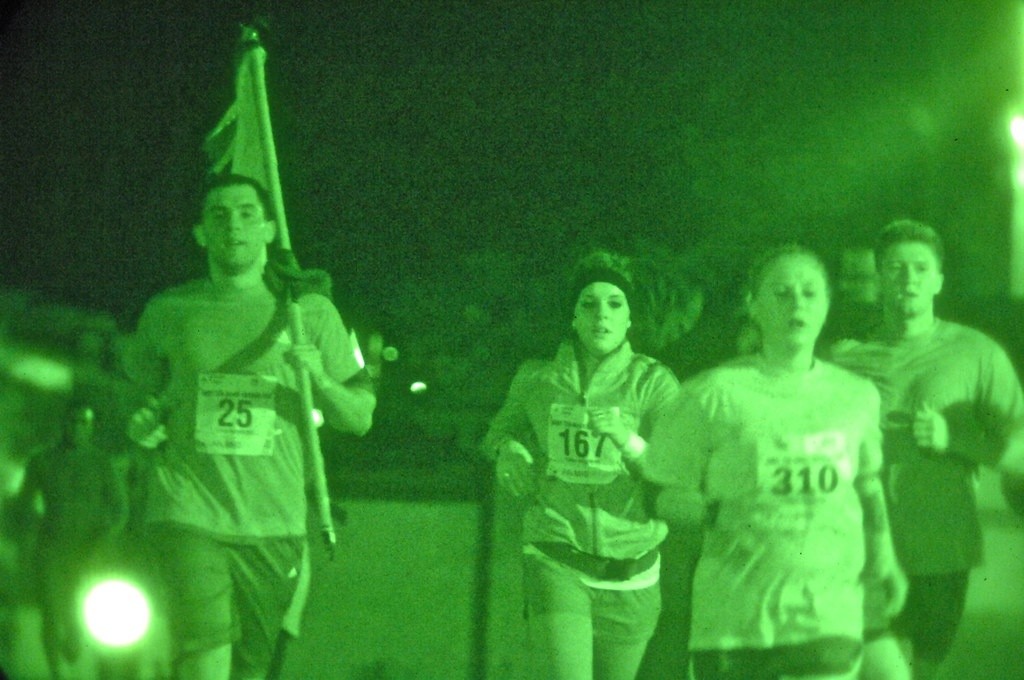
[[816, 240, 883, 367], [643, 241, 909, 680], [11, 404, 130, 679], [117, 172, 377, 680], [826, 218, 1024, 679], [480, 254, 683, 680]]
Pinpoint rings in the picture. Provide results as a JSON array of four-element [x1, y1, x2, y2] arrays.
[[597, 413, 605, 420], [503, 472, 511, 477]]
